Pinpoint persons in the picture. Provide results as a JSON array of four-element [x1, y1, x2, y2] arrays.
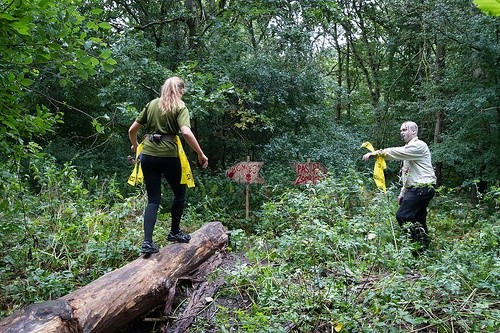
[[361, 120, 438, 256], [128, 76, 209, 253]]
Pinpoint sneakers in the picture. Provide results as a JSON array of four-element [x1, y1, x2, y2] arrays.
[[140, 240, 159, 253], [166, 230, 191, 243]]
